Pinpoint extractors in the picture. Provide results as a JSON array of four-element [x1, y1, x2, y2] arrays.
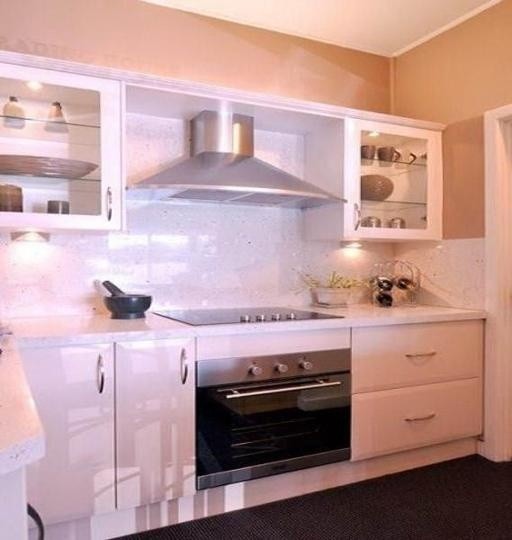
[[126, 109, 348, 210]]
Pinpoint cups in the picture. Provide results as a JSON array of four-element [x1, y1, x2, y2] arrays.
[[362, 216, 382, 228], [390, 217, 406, 229], [361, 142, 417, 169]]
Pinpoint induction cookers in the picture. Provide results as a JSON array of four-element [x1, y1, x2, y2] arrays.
[[151, 306, 345, 326]]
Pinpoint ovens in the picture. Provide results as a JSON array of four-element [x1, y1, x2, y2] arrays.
[[195, 348, 352, 491]]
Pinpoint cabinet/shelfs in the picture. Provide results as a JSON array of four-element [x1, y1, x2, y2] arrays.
[[349, 321, 486, 485], [23, 338, 197, 540], [0, 51, 128, 236], [301, 107, 449, 243]]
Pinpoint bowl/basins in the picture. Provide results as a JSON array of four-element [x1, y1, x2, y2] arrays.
[[103, 294, 152, 320]]
